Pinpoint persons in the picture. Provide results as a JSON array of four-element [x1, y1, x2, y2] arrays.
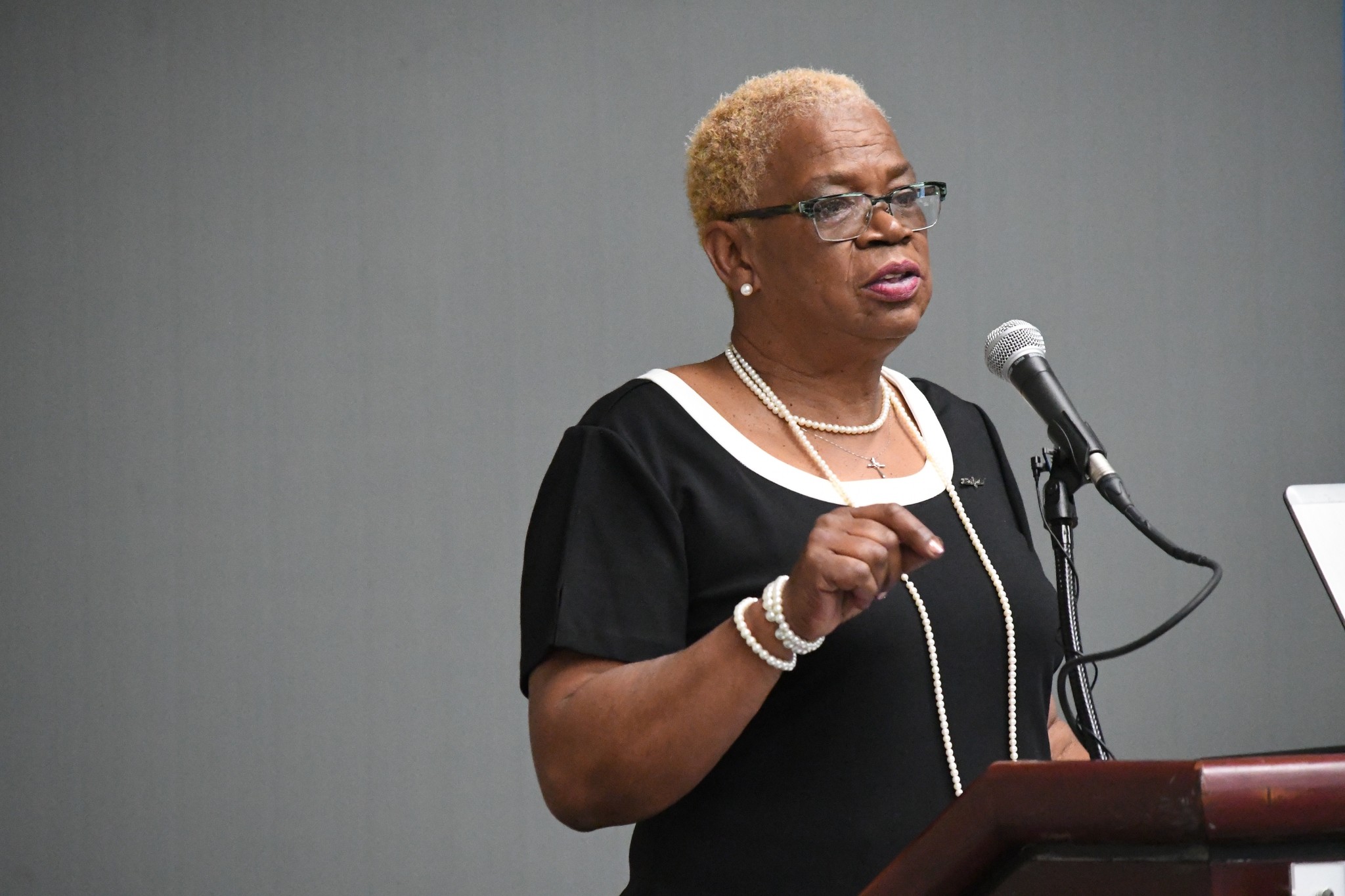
[[518, 67, 1099, 896]]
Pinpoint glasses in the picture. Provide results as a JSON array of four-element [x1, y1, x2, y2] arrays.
[[722, 181, 948, 243]]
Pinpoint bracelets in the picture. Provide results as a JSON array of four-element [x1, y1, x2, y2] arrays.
[[733, 575, 825, 671]]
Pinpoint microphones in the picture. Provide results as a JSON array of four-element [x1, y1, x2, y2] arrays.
[[984, 320, 1134, 512]]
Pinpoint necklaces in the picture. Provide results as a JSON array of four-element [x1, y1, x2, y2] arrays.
[[725, 341, 1019, 796], [724, 345, 893, 479]]
